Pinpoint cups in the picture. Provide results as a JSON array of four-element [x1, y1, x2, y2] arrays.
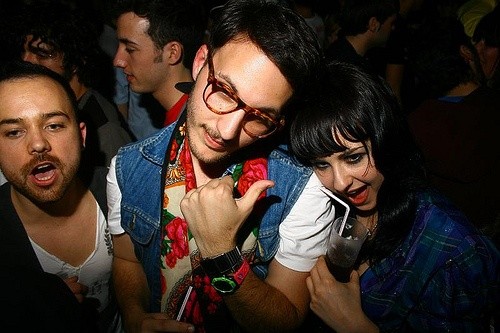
[[325, 217, 369, 283]]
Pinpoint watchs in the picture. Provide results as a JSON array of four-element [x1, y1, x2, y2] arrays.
[[202, 245, 245, 277], [212, 261, 249, 294]]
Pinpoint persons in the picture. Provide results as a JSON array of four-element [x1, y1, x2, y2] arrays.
[[102, 8, 337, 333], [329, 0, 500, 186], [19, 12, 109, 206], [283, 72, 500, 333], [0, 60, 134, 333]]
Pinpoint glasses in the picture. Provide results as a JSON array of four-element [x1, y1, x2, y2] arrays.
[[202, 51, 279, 139]]
